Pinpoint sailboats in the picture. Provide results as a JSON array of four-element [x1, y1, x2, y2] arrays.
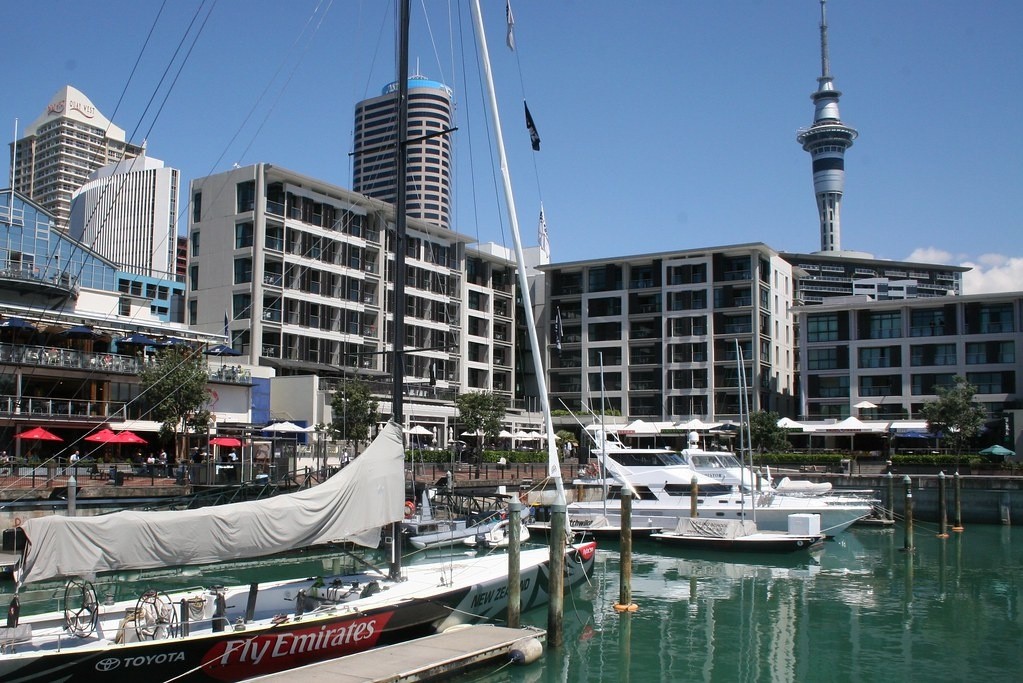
[[0, 0, 599, 683]]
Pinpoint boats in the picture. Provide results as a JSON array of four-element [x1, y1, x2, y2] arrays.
[[502, 267, 879, 554], [403, 429, 531, 551]]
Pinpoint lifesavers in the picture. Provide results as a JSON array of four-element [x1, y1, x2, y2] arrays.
[[404, 500, 416, 519], [586, 462, 599, 476]]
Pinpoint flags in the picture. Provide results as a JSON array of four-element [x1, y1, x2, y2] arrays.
[[525, 99, 540, 151], [536, 202, 550, 259], [555, 308, 564, 351], [506, 0, 515, 51], [225, 313, 229, 341]]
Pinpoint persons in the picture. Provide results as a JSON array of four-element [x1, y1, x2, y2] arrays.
[[288, 443, 294, 458], [221, 365, 242, 383], [0, 450, 9, 467], [103, 450, 112, 464], [95, 354, 112, 371], [193, 449, 203, 464], [83, 452, 94, 462], [340, 449, 349, 467], [228, 449, 239, 463], [25, 450, 41, 467], [143, 353, 157, 371], [38, 344, 60, 365], [134, 448, 167, 477], [69, 450, 80, 468], [567, 441, 572, 457]]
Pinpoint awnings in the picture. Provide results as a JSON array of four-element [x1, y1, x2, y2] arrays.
[[891, 420, 954, 439], [979, 444, 1016, 457]]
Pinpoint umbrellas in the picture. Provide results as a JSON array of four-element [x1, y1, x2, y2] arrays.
[[202, 345, 242, 365], [55, 327, 104, 354], [774, 418, 806, 442], [85, 429, 148, 455], [114, 333, 158, 356], [209, 438, 247, 449], [825, 417, 871, 440], [620, 418, 721, 445], [157, 337, 196, 355], [0, 319, 38, 343], [260, 422, 342, 463], [853, 400, 879, 412], [403, 424, 432, 445], [462, 427, 562, 450], [14, 428, 64, 447]]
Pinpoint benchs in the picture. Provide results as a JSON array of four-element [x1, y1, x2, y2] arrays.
[[84, 462, 138, 482], [799, 465, 827, 473]]
